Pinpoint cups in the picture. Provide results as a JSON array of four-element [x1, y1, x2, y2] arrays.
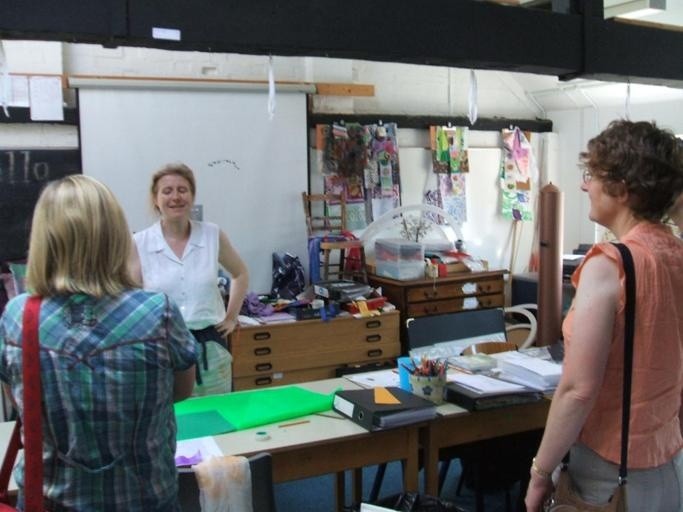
[[398, 357, 445, 405]]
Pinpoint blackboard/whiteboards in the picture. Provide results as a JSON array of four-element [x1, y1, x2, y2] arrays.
[[0, 149, 82, 275]]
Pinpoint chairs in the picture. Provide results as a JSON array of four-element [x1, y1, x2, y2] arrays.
[[303, 190, 363, 280]]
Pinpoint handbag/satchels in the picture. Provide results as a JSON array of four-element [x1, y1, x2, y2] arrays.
[[540, 465, 628, 512], [0, 503, 18, 512]]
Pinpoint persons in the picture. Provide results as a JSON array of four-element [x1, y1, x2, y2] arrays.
[[127, 162, 250, 398], [0, 171, 199, 511], [523, 116, 682, 512], [663, 135, 683, 242]]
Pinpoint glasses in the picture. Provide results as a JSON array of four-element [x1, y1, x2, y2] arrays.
[[583, 170, 593, 184]]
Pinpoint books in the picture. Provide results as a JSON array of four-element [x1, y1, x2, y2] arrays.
[[439, 351, 563, 413], [258, 310, 298, 326]]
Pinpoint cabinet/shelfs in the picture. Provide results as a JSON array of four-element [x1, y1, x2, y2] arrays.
[[367, 273, 505, 321], [228, 309, 401, 393]]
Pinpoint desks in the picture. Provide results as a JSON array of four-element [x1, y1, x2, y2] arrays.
[[341, 346, 567, 499], [0, 377, 428, 507]]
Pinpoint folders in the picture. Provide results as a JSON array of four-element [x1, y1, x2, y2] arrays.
[[444, 372, 545, 412]]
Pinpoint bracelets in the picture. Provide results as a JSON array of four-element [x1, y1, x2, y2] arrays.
[[530, 456, 553, 481]]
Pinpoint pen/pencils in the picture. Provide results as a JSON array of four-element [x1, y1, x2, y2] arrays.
[[401, 351, 449, 376]]
